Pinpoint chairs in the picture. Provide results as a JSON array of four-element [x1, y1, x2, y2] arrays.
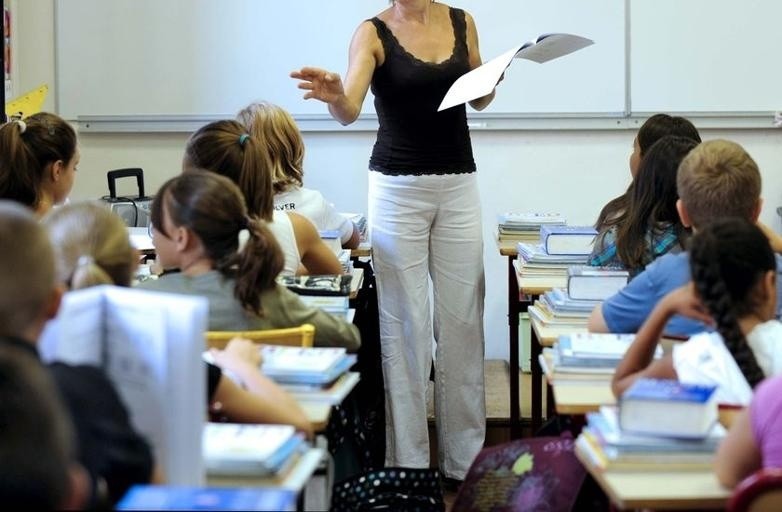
[[206, 323, 315, 353], [728, 468, 782, 512], [719, 402, 745, 432]]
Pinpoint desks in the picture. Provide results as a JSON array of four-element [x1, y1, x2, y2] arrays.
[[492, 230, 520, 441], [551, 379, 619, 438], [573, 441, 735, 512], [295, 401, 332, 432], [351, 247, 371, 257], [336, 268, 365, 299], [346, 308, 356, 324], [537, 353, 558, 436], [530, 307, 588, 436], [204, 444, 304, 488], [515, 261, 566, 439]]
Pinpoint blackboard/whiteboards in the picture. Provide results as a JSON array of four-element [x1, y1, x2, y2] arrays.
[[53, 0, 782, 134]]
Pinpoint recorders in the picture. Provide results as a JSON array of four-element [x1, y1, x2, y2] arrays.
[[100, 169, 155, 228]]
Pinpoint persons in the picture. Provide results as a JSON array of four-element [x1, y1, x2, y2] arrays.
[[587, 113, 781, 489], [0, 99, 362, 509], [288, 1, 506, 492]]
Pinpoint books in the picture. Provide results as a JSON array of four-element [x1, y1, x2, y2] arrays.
[[494, 208, 665, 384], [437, 32, 596, 111]]
